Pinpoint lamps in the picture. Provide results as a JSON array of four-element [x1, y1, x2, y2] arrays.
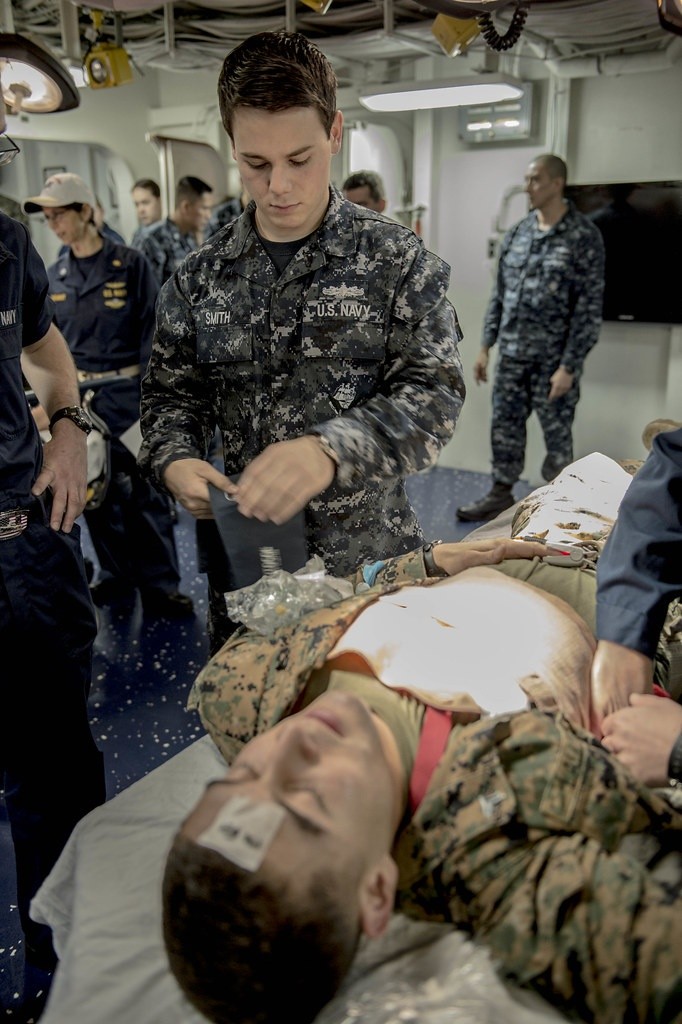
[[300, 0, 333, 15], [657, 0, 682, 41], [81, 9, 134, 91], [430, 10, 493, 60], [0, 29, 82, 116], [356, 70, 529, 118]]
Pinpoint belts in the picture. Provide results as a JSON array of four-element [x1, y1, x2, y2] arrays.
[[75, 363, 140, 382]]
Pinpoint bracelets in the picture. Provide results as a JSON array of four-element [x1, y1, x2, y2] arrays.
[[667, 729, 682, 790]]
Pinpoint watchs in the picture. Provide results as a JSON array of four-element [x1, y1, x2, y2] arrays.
[[308, 430, 339, 466], [423, 538, 447, 578], [48, 406, 92, 436]]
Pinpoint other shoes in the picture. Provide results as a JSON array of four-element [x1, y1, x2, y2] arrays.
[[90, 580, 132, 605], [456, 490, 514, 520], [142, 592, 194, 612]]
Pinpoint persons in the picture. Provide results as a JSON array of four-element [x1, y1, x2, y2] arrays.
[[0, 81, 107, 992], [23, 172, 194, 623], [137, 31, 467, 659], [129, 179, 160, 249], [341, 170, 386, 213], [161, 418, 682, 1024], [139, 175, 213, 289], [456, 154, 606, 522]]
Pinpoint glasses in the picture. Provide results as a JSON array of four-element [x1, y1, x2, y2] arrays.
[[39, 207, 73, 225]]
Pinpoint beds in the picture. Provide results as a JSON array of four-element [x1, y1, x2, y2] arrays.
[[26, 496, 572, 1024]]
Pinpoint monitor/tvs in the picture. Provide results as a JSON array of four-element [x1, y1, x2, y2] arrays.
[[562, 180, 682, 325]]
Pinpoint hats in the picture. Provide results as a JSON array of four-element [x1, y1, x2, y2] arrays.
[[22, 173, 94, 213]]
